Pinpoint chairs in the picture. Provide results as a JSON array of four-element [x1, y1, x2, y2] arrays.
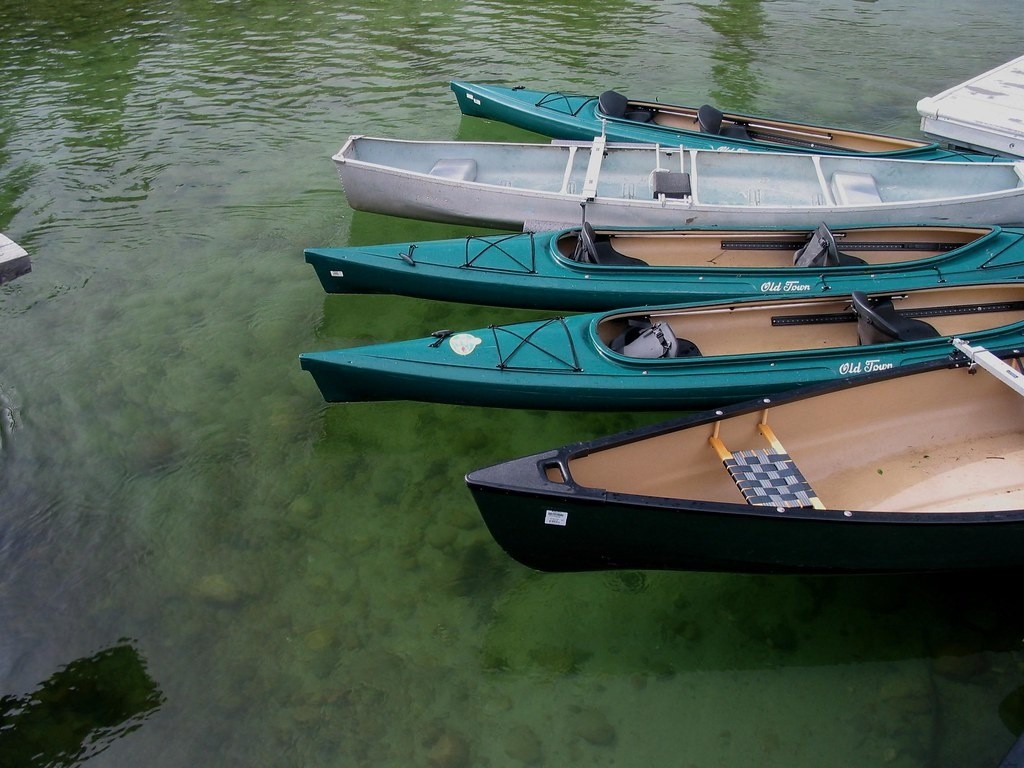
[[798, 224, 872, 267], [849, 288, 940, 347], [696, 104, 754, 141], [598, 91, 656, 124], [621, 323, 701, 361], [575, 225, 648, 268]]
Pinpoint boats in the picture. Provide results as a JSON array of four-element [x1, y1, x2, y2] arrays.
[[300, 79, 1024, 573]]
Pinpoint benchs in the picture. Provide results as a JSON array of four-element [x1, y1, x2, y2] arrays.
[[708, 426, 828, 509], [832, 169, 879, 204], [431, 157, 477, 183]]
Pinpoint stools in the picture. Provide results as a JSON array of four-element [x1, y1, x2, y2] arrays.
[[650, 170, 695, 196]]
